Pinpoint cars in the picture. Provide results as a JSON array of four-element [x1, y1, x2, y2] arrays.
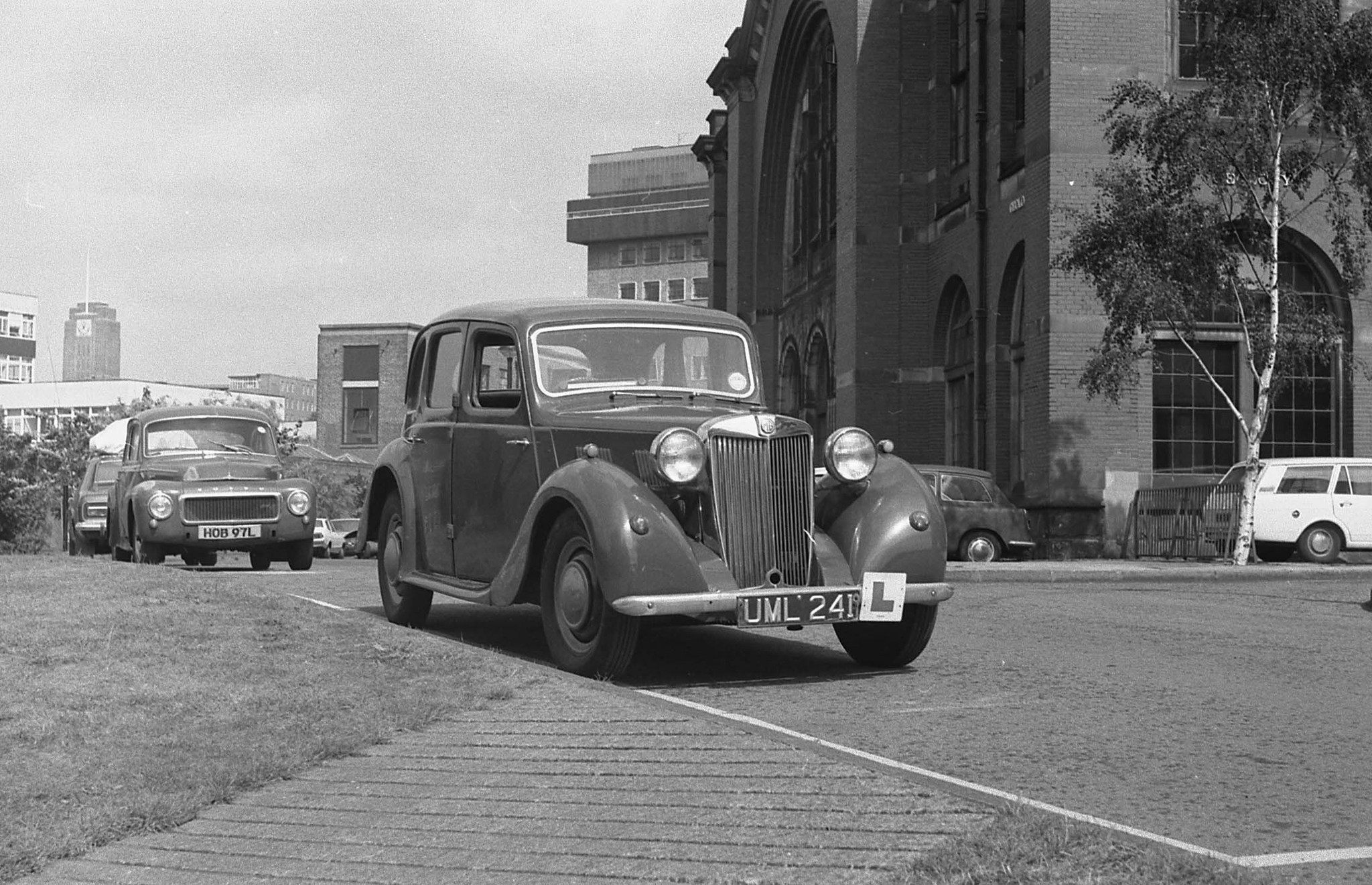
[[69, 407, 361, 571], [1202, 458, 1372, 563], [351, 298, 955, 679], [911, 464, 1036, 563]]
